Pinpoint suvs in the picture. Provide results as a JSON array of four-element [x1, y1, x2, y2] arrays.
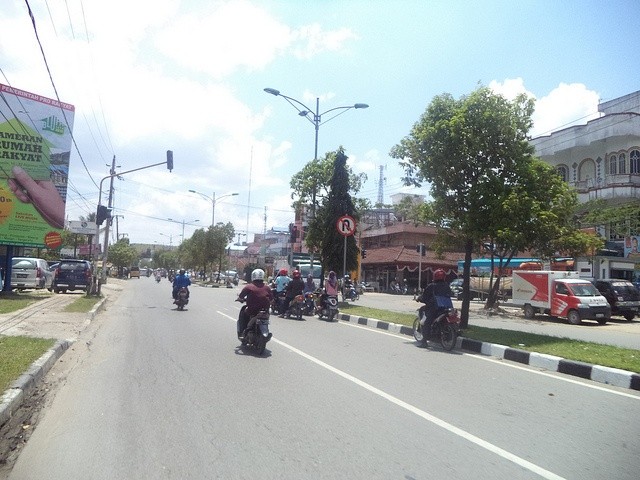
[[52, 259, 94, 294]]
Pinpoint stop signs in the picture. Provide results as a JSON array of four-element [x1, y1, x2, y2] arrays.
[[336, 216, 356, 236]]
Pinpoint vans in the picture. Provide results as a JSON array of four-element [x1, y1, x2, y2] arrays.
[[592, 279, 640, 322], [450, 278, 465, 300], [295, 264, 325, 290]]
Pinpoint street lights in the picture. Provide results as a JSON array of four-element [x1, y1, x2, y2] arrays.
[[159, 232, 183, 250], [262, 87, 370, 277], [168, 217, 200, 242], [155, 241, 173, 248], [189, 188, 240, 224]]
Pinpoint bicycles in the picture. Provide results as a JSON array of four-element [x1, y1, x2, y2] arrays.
[[354, 284, 360, 301]]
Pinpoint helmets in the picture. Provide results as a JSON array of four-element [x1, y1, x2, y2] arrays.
[[292, 270, 301, 279], [180, 268, 185, 275], [280, 268, 288, 276], [307, 274, 312, 281], [433, 270, 446, 280], [251, 269, 265, 282]]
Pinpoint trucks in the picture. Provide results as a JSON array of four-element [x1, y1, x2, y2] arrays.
[[513, 270, 611, 324]]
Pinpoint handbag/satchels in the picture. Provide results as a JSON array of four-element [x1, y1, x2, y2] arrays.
[[279, 277, 289, 290], [293, 281, 302, 295]]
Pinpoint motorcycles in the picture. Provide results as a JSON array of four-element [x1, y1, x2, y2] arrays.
[[171, 285, 190, 311], [314, 288, 339, 321], [303, 291, 315, 316], [410, 295, 464, 352], [279, 283, 303, 319], [401, 284, 409, 295], [392, 282, 400, 295], [269, 282, 280, 313], [235, 298, 273, 357], [343, 284, 356, 302], [155, 273, 161, 283]]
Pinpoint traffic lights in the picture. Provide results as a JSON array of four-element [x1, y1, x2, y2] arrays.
[[167, 151, 173, 172], [96, 206, 112, 225]]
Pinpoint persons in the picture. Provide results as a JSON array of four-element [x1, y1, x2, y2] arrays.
[[273, 269, 280, 288], [343, 274, 353, 289], [8, 165, 64, 230], [318, 270, 339, 311], [277, 269, 307, 318], [415, 269, 453, 349], [272, 268, 293, 315], [237, 268, 275, 334], [627, 238, 640, 259], [172, 268, 191, 304], [156, 268, 161, 277], [303, 274, 316, 298]]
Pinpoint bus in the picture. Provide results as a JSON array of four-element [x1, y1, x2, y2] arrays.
[[457, 258, 576, 300], [130, 266, 140, 279]]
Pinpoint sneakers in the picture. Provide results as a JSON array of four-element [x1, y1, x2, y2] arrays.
[[416, 343, 428, 348]]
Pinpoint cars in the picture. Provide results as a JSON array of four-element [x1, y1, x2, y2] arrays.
[[198, 272, 207, 279], [157, 270, 166, 276], [210, 272, 225, 280], [9, 256, 49, 289], [47, 262, 59, 289]]
